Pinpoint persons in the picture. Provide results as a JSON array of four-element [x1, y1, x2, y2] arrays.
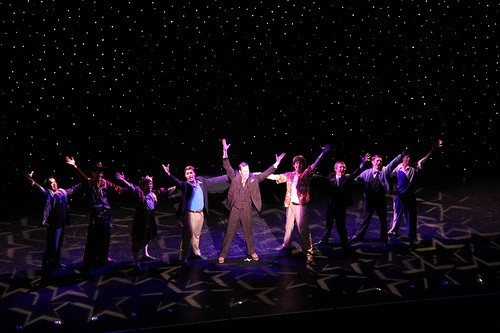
[[351, 150, 407, 251], [265, 142, 332, 264], [310, 151, 371, 254], [116, 171, 168, 273], [66, 155, 132, 278], [25, 169, 84, 282], [387, 139, 443, 250], [219, 138, 286, 263], [162, 161, 231, 269]]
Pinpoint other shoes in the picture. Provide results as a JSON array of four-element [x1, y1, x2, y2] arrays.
[[179, 261, 189, 268], [251, 253, 259, 261], [134, 263, 142, 272], [106, 258, 116, 263], [387, 230, 397, 236], [307, 253, 314, 262], [276, 245, 288, 250], [337, 242, 349, 247], [141, 256, 156, 260], [218, 257, 224, 264], [349, 235, 358, 242], [60, 264, 67, 267], [189, 254, 201, 260], [313, 239, 329, 246]]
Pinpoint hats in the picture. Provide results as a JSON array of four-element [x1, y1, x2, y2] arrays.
[[89, 161, 108, 174]]
